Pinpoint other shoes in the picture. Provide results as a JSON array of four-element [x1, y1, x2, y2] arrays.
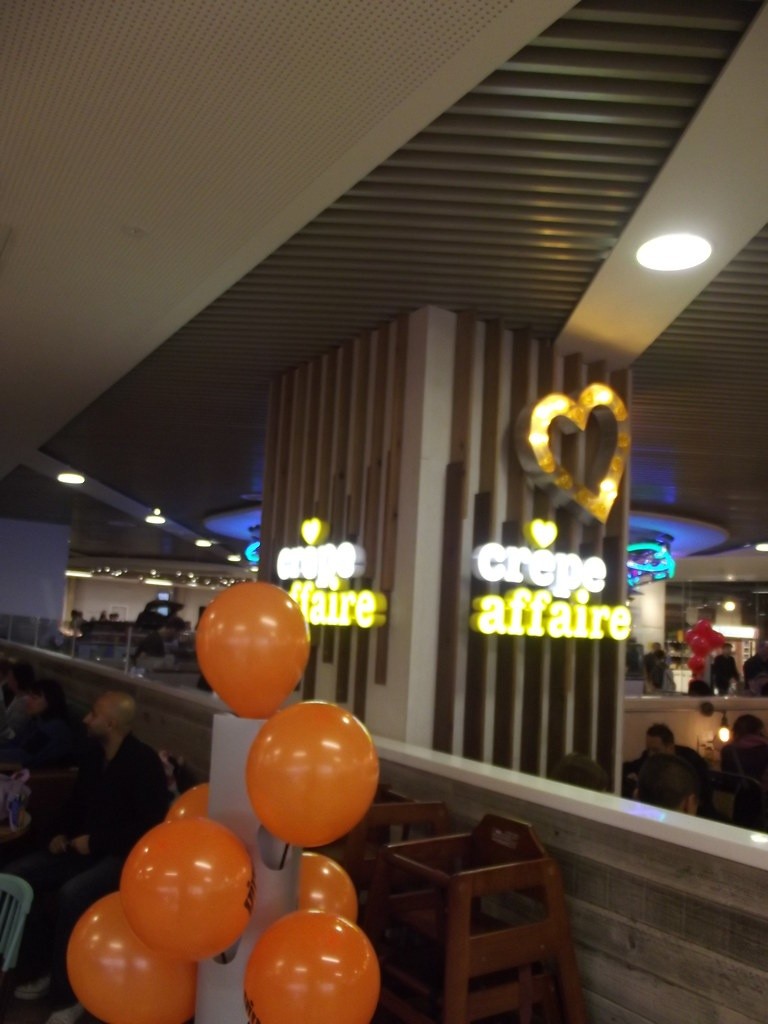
[[45, 1003, 85, 1024], [13, 971, 55, 1000]]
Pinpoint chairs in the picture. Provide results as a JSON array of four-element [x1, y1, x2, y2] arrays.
[[0, 873, 33, 989], [303, 784, 588, 1024], [696, 768, 763, 827]]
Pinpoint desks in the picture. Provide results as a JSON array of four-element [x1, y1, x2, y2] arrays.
[[0, 809, 32, 843]]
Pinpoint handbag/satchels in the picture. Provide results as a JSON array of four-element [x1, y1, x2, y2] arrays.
[[0, 767, 31, 832]]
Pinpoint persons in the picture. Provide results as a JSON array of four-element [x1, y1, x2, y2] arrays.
[[0, 658, 71, 768], [129, 616, 187, 665], [720, 714, 768, 779], [645, 642, 669, 687], [713, 642, 740, 695], [740, 640, 768, 697], [70, 610, 86, 630], [0, 690, 170, 1024], [623, 724, 714, 817]]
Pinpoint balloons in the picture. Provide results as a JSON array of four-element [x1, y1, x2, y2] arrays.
[[165, 784, 210, 821], [195, 581, 312, 719], [247, 700, 380, 848], [120, 817, 256, 965], [244, 909, 381, 1024], [686, 619, 724, 676], [67, 891, 198, 1024], [297, 852, 358, 923]]
